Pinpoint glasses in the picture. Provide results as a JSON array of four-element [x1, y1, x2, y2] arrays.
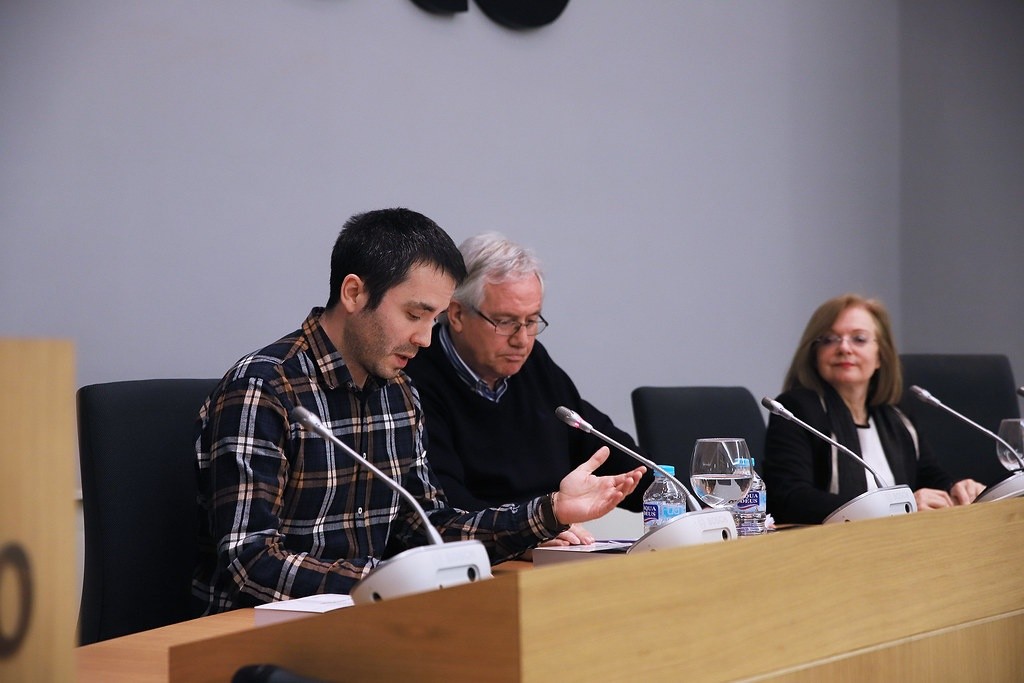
[[472, 308, 548, 336], [815, 332, 879, 347]]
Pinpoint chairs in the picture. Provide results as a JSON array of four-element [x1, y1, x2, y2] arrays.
[[900, 353, 1020, 493], [630, 385, 767, 482], [75, 377, 221, 647]]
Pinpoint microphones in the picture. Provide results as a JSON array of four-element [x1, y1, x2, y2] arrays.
[[761, 396, 917, 525], [554, 407, 738, 556], [290, 406, 495, 607], [908, 383, 1024, 504]]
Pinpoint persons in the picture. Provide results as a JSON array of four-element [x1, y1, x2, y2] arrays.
[[190, 208, 646, 622], [401, 233, 658, 561], [761, 294, 987, 525]]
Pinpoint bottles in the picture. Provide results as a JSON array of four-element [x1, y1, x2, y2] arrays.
[[642, 464, 687, 534], [732, 457, 767, 537]]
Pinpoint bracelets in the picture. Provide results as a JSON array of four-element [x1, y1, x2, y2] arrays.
[[541, 492, 571, 533]]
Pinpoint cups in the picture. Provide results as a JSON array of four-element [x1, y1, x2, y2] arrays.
[[996, 419, 1024, 474], [690, 437, 753, 512]]
